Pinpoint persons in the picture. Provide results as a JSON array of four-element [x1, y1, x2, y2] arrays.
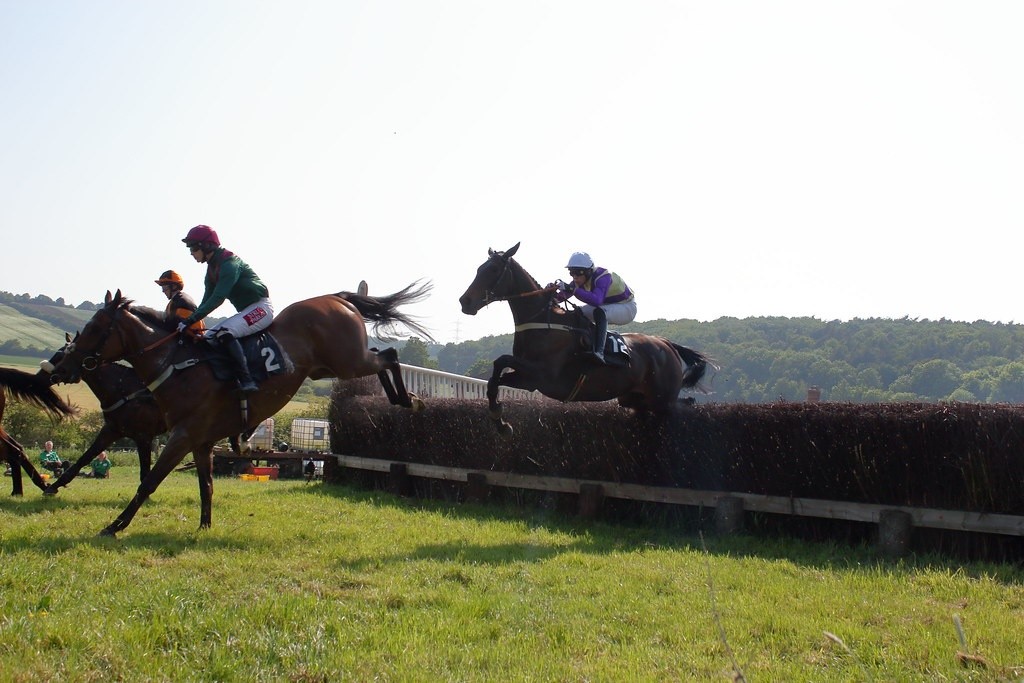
[[40, 441, 111, 479], [154, 270, 205, 335], [545, 252, 637, 367], [176, 225, 274, 391], [305, 457, 314, 474]]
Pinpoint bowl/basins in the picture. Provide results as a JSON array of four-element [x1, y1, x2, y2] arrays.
[[40, 474, 50, 479], [239, 467, 279, 482]]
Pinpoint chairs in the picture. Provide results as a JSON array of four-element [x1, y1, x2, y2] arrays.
[[39, 452, 56, 478]]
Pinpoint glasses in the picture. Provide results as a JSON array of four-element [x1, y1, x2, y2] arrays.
[[190, 244, 201, 252], [570, 269, 585, 276]]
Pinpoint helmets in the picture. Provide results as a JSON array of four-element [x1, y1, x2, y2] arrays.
[[155, 270, 183, 288], [564, 252, 592, 268], [182, 226, 220, 246]]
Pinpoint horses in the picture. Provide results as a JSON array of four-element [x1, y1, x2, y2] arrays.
[[459, 242, 720, 442], [0, 277, 437, 538]]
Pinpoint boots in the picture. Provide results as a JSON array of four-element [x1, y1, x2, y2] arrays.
[[226, 339, 260, 391], [592, 320, 608, 365]]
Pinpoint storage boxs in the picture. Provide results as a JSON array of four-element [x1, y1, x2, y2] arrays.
[[241, 466, 280, 482]]
